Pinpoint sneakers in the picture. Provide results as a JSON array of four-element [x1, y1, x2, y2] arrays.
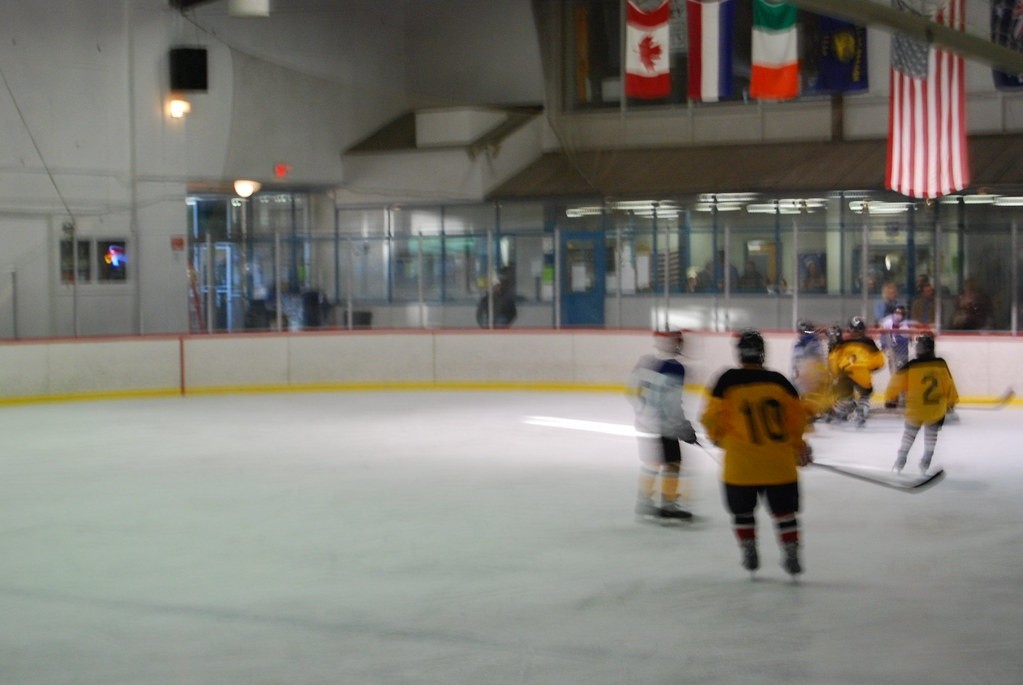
[[782, 557, 800, 579], [634, 499, 659, 521], [657, 502, 692, 523], [893, 458, 905, 474], [743, 549, 759, 579], [919, 459, 930, 476]]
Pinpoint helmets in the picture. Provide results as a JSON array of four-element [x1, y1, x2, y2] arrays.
[[913, 335, 934, 354], [849, 319, 865, 333], [893, 305, 906, 317], [798, 321, 813, 337], [655, 330, 684, 353], [827, 327, 842, 343], [737, 332, 764, 357]]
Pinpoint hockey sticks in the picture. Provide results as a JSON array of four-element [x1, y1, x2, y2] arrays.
[[809, 461, 947, 494], [696, 439, 722, 467], [954, 389, 1016, 410]]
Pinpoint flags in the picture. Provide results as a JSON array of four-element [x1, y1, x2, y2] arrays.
[[805, 14, 870, 96], [685, 0, 736, 102], [751, 0, 800, 100], [623, 0, 672, 102], [989, 0, 1023, 93], [886, 0, 970, 198]]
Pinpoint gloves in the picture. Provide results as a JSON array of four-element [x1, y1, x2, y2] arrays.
[[676, 421, 697, 444], [797, 443, 812, 467]]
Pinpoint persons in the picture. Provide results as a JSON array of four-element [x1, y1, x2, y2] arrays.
[[476, 279, 518, 327], [882, 335, 960, 473], [791, 307, 918, 426], [626, 327, 697, 522], [700, 331, 811, 583], [801, 261, 827, 291], [852, 271, 994, 330], [687, 251, 767, 293]]
